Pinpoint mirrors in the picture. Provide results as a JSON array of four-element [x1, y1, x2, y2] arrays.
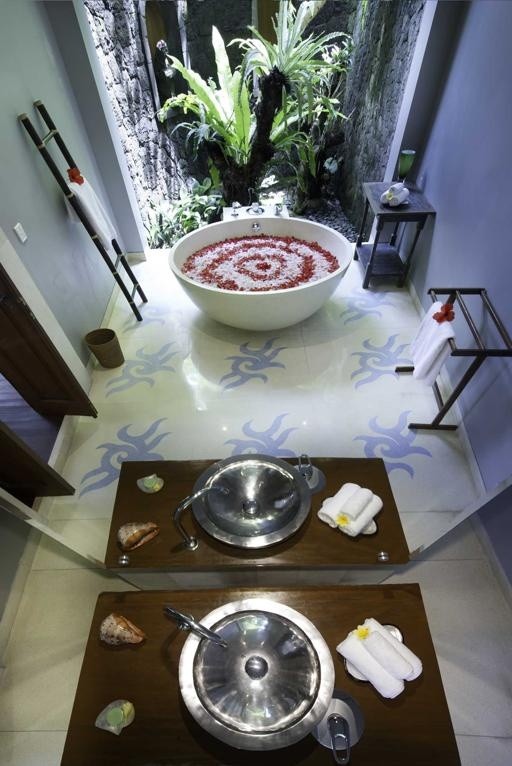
[[0, 0, 510, 588]]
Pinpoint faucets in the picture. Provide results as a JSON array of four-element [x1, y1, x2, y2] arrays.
[[168, 484, 233, 551], [161, 603, 232, 652], [249, 202, 263, 217]]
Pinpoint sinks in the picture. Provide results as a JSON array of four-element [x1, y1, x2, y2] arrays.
[[189, 456, 313, 547], [176, 596, 337, 751]]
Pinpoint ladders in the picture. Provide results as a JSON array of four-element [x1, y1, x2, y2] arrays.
[[18, 100, 148, 321]]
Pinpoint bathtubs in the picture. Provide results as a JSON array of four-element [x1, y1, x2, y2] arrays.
[[167, 215, 355, 333]]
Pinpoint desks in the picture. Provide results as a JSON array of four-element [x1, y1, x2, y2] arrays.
[[351, 179, 436, 290]]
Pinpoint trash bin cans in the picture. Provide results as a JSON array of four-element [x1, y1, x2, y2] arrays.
[[85, 328, 125, 369]]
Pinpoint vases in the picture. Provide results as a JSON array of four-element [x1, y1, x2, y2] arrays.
[[397, 149, 416, 183]]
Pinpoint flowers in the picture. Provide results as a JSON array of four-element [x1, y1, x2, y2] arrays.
[[66, 167, 84, 185], [432, 304, 455, 324]]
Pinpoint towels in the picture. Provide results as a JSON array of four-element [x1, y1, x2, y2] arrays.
[[338, 614, 425, 700], [319, 480, 384, 536], [379, 181, 411, 205], [410, 301, 457, 385], [64, 173, 116, 248]]
